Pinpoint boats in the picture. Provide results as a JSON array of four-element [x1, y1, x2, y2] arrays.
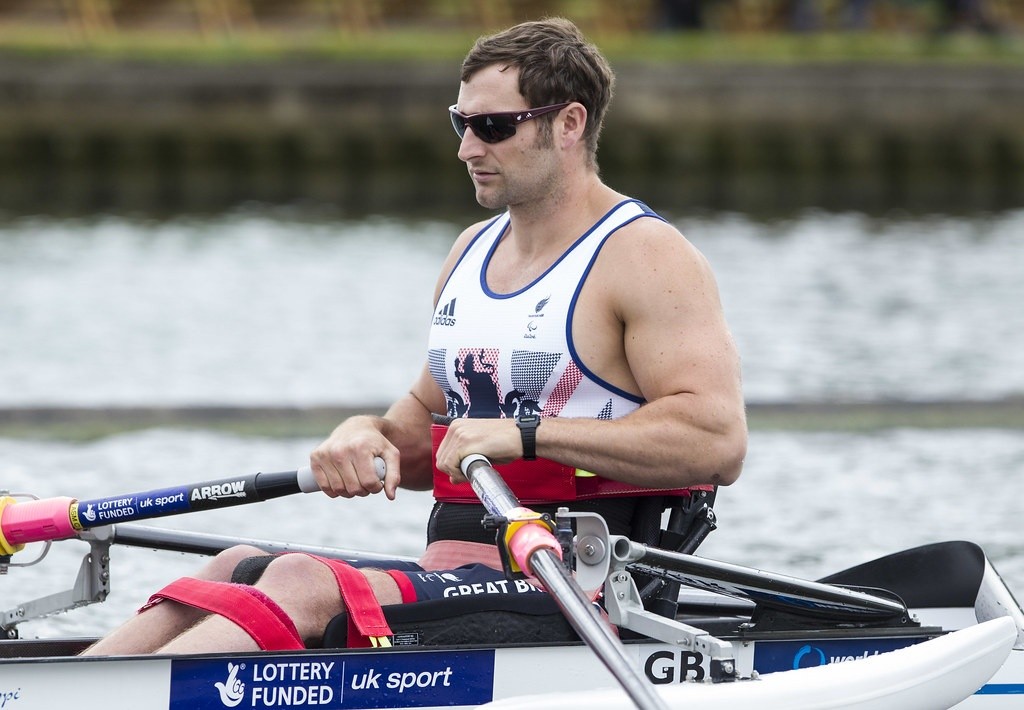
[[0, 519, 1024, 710]]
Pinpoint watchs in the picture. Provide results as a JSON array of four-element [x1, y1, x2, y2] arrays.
[[516, 415, 540, 459]]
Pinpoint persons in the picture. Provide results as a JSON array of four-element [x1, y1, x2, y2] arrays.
[[76, 18, 749, 656]]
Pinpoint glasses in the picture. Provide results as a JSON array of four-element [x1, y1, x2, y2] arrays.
[[447, 102, 572, 144]]
